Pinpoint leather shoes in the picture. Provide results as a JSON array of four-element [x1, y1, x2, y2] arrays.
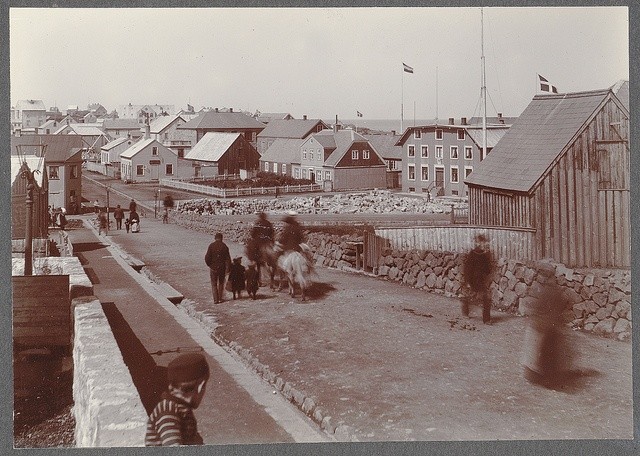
[[483, 321, 492, 326], [463, 315, 470, 319], [219, 299, 225, 303], [214, 301, 218, 304]]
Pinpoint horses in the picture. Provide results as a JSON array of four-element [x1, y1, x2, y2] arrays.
[[277, 249, 317, 301], [244, 237, 278, 289]]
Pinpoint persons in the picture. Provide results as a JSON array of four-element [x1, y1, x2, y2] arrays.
[[144, 354, 211, 449], [461, 234, 499, 325], [114, 205, 124, 230], [520, 264, 583, 392], [93, 200, 101, 214], [124, 218, 130, 234], [205, 233, 233, 304], [227, 257, 247, 300], [245, 261, 263, 300], [249, 212, 275, 265], [129, 199, 137, 212], [97, 212, 109, 235], [129, 208, 140, 233], [426, 191, 431, 203], [268, 215, 301, 277], [162, 208, 168, 224]]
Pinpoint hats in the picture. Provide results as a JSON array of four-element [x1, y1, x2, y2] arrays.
[[118, 205, 120, 208]]
